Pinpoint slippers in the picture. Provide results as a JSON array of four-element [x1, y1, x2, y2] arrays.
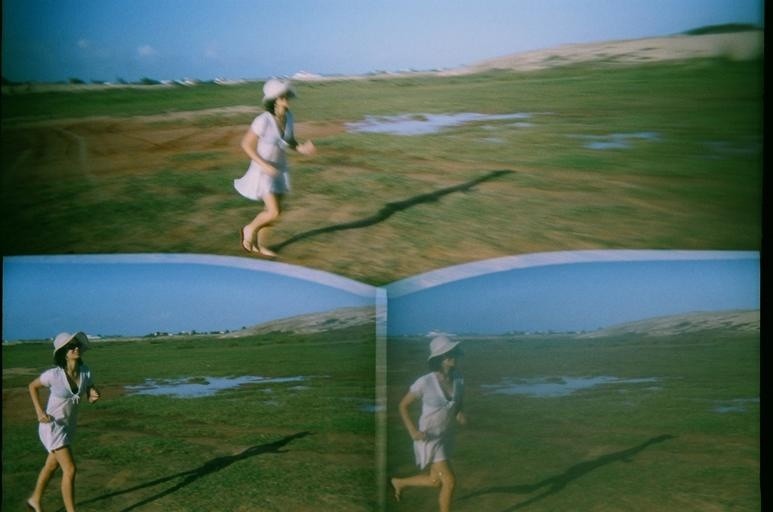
[[240, 225, 252, 254], [389, 477, 401, 504]]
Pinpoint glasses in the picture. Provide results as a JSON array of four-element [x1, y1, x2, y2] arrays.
[[67, 342, 82, 350]]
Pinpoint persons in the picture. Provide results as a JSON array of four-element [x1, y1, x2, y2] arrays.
[[27, 331, 100, 512], [390, 335, 468, 512], [233, 78, 315, 257]]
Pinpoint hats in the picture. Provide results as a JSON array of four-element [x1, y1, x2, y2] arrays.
[[52, 331, 91, 358], [426, 335, 462, 362], [263, 78, 297, 106]]
